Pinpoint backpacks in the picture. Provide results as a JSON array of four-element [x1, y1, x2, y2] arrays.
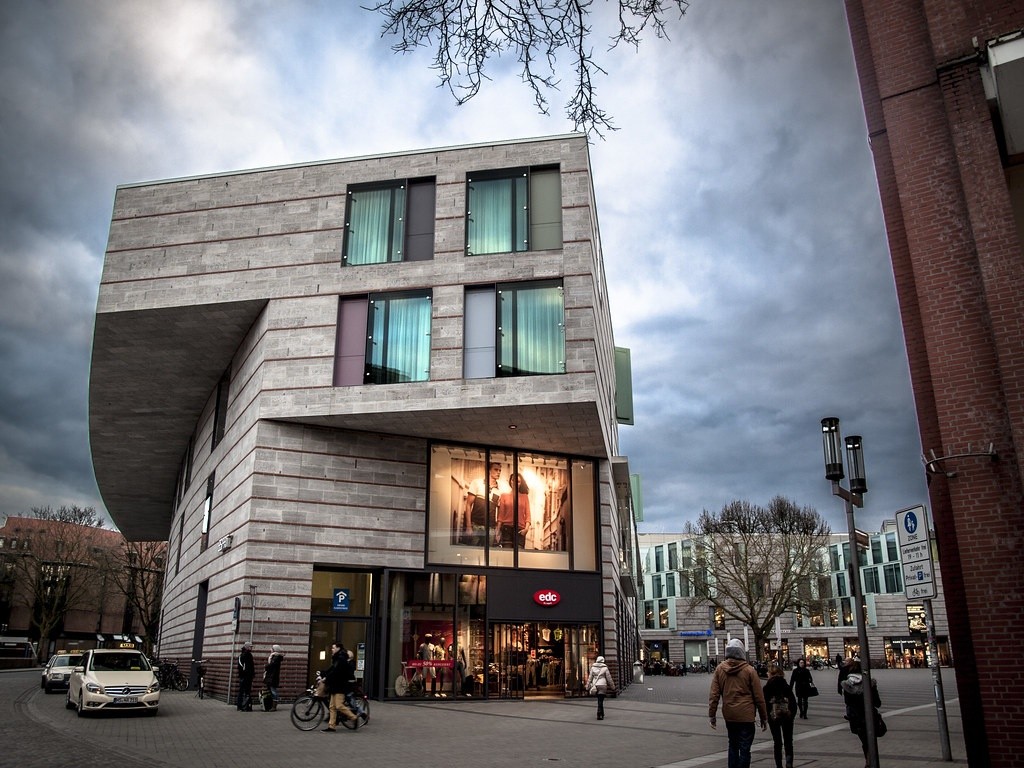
[[770, 696, 790, 720]]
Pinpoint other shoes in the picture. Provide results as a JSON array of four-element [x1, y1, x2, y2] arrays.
[[320, 728, 336, 733]]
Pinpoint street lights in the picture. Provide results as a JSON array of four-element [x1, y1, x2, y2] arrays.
[[820, 417, 881, 768]]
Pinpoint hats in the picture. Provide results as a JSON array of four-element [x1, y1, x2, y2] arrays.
[[245, 641, 254, 648], [596, 657, 604, 662], [273, 645, 281, 651]]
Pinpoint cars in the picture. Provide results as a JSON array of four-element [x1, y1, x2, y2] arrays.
[[64, 643, 161, 717], [41, 650, 85, 694]]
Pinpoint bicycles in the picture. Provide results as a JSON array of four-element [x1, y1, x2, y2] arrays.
[[191, 659, 210, 699], [290, 671, 370, 732], [151, 659, 189, 691], [393, 661, 426, 697], [687, 663, 715, 673], [805, 661, 824, 671]]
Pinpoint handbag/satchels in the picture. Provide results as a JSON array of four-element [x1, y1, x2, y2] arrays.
[[258, 688, 273, 711], [590, 686, 597, 695], [808, 682, 819, 697]]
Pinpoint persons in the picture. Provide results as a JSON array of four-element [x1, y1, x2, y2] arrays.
[[465, 461, 531, 549], [262, 644, 284, 712], [789, 658, 812, 719], [889, 650, 948, 668], [507, 629, 523, 651], [749, 649, 833, 677], [708, 638, 767, 768], [763, 665, 798, 768], [316, 641, 370, 733], [418, 634, 472, 697], [236, 642, 254, 712], [841, 661, 882, 768], [585, 656, 615, 720], [644, 655, 717, 678], [836, 650, 861, 721]]
